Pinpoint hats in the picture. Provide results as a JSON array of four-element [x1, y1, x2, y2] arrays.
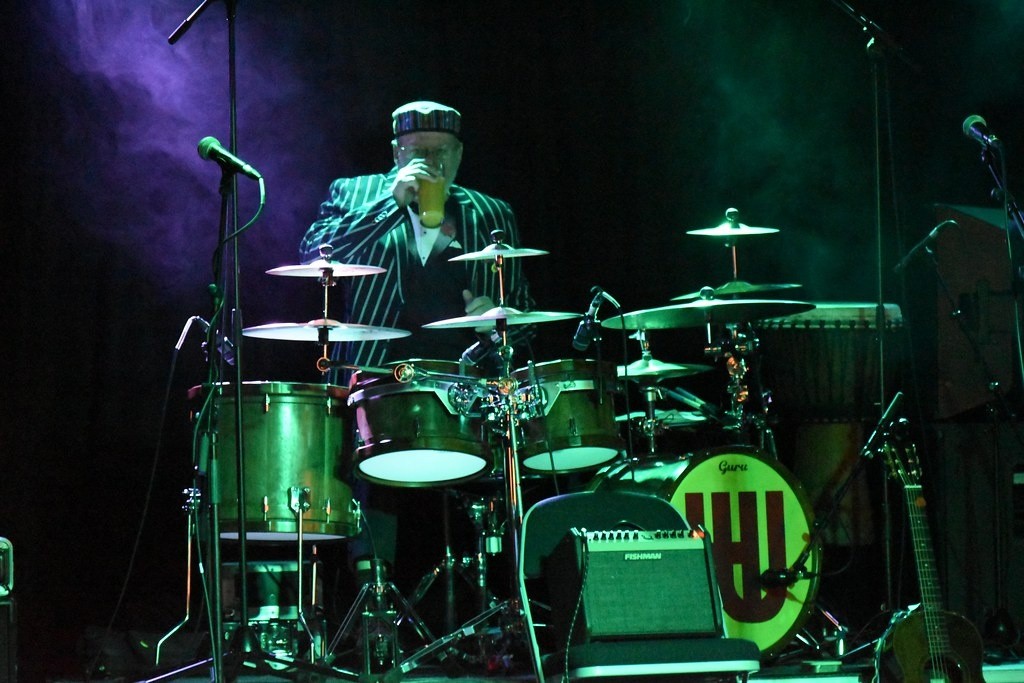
[[391, 100, 463, 138]]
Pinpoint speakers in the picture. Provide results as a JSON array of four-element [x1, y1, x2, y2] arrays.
[[550, 527, 723, 641], [788, 417, 879, 550], [0, 597, 20, 683]]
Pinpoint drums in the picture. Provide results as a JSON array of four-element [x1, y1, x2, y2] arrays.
[[345, 357, 499, 488], [504, 359, 628, 476], [187, 380, 364, 541], [587, 445, 824, 661]]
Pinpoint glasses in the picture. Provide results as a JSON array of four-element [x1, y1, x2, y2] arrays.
[[396, 142, 460, 160]]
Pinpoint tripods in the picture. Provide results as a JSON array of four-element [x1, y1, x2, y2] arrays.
[[138, 255, 552, 683], [926, 244, 1024, 662]]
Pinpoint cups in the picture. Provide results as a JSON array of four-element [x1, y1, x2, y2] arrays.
[[418, 161, 445, 227]]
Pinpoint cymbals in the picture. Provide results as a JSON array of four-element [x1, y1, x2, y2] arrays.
[[447, 243, 549, 261], [601, 280, 817, 329], [242, 318, 412, 341], [264, 259, 387, 277], [420, 306, 585, 329], [614, 409, 710, 427], [616, 359, 712, 380], [686, 222, 780, 246]]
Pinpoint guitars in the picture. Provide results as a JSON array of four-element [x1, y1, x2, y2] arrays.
[[879, 414, 990, 683]]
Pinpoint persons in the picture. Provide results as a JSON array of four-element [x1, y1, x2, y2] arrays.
[[299, 100, 537, 636]]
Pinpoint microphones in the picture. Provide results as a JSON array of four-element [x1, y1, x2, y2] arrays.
[[963, 114, 1002, 147], [895, 219, 950, 275], [658, 383, 722, 416], [196, 317, 233, 366], [760, 568, 817, 588], [197, 136, 261, 180], [572, 292, 604, 352], [462, 324, 527, 366]]
